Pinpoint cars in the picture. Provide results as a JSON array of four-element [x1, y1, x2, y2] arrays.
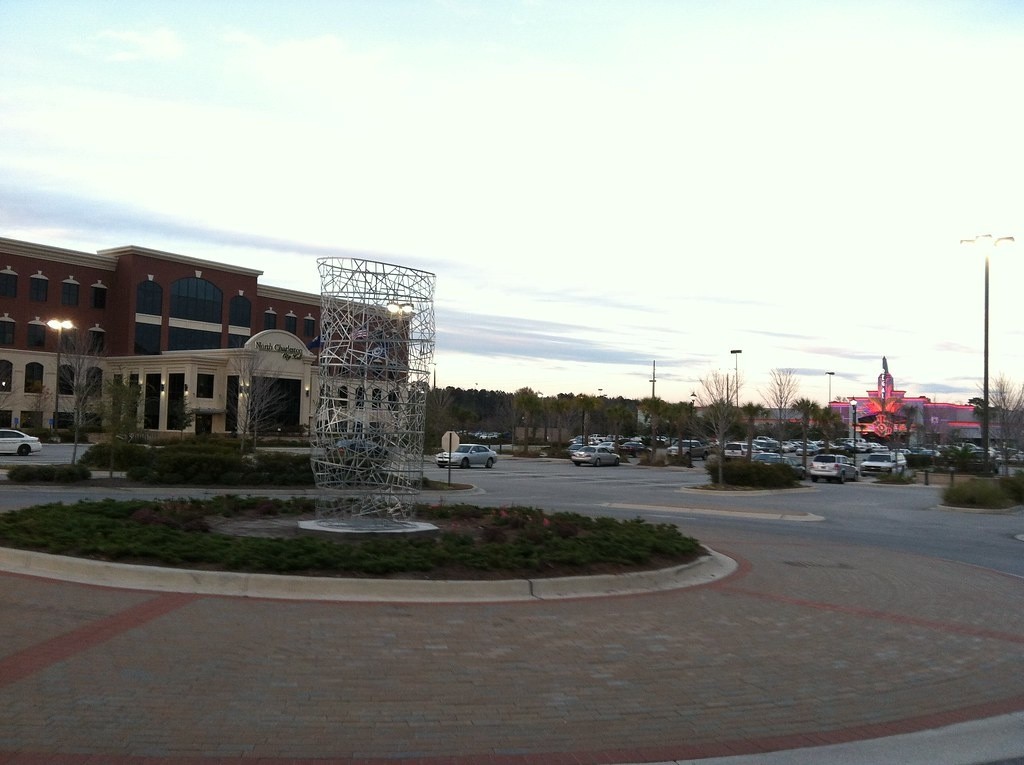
[[0, 429, 43, 456], [860, 452, 907, 477], [456, 430, 502, 440], [569, 433, 668, 458], [571, 446, 621, 467], [752, 453, 806, 480], [435, 443, 498, 469], [810, 453, 859, 484], [756, 436, 1024, 461]]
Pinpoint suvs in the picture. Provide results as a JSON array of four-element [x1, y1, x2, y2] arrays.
[[723, 442, 764, 457]]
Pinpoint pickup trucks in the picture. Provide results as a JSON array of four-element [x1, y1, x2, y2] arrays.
[[666, 439, 711, 461]]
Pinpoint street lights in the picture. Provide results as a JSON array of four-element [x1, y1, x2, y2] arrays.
[[47, 318, 74, 439], [961, 234, 1015, 474], [849, 395, 858, 467], [385, 301, 415, 478]]
